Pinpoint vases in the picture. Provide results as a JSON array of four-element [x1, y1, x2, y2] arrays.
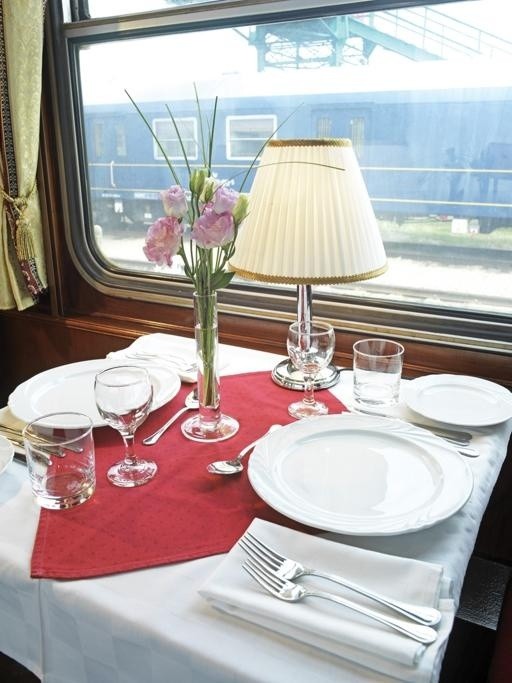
[[176, 289, 243, 447]]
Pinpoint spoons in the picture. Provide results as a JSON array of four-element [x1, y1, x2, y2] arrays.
[[142, 387, 199, 446], [206, 423, 283, 474]]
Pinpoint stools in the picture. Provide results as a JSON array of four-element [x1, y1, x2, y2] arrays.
[[438, 553, 512, 683]]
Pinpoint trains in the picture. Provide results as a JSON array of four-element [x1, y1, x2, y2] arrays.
[[81, 89, 512, 237]]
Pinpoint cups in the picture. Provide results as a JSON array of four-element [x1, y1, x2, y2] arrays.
[[22, 410, 96, 509], [352, 338, 404, 414]]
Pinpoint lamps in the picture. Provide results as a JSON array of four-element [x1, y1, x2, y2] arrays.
[[221, 133, 397, 395]]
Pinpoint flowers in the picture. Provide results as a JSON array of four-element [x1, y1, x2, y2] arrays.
[[119, 83, 347, 409]]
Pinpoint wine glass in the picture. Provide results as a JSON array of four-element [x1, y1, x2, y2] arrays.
[[285, 319, 336, 419], [94, 367, 157, 487]]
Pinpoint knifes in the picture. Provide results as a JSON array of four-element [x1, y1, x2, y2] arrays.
[[0, 422, 86, 465], [353, 406, 472, 440]]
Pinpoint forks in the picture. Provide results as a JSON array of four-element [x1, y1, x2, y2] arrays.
[[235, 530, 443, 626], [242, 556, 438, 648]]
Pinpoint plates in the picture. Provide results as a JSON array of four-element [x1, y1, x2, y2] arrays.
[[8, 358, 181, 428], [247, 412, 474, 538], [0, 434, 14, 475], [403, 373, 512, 426]]
